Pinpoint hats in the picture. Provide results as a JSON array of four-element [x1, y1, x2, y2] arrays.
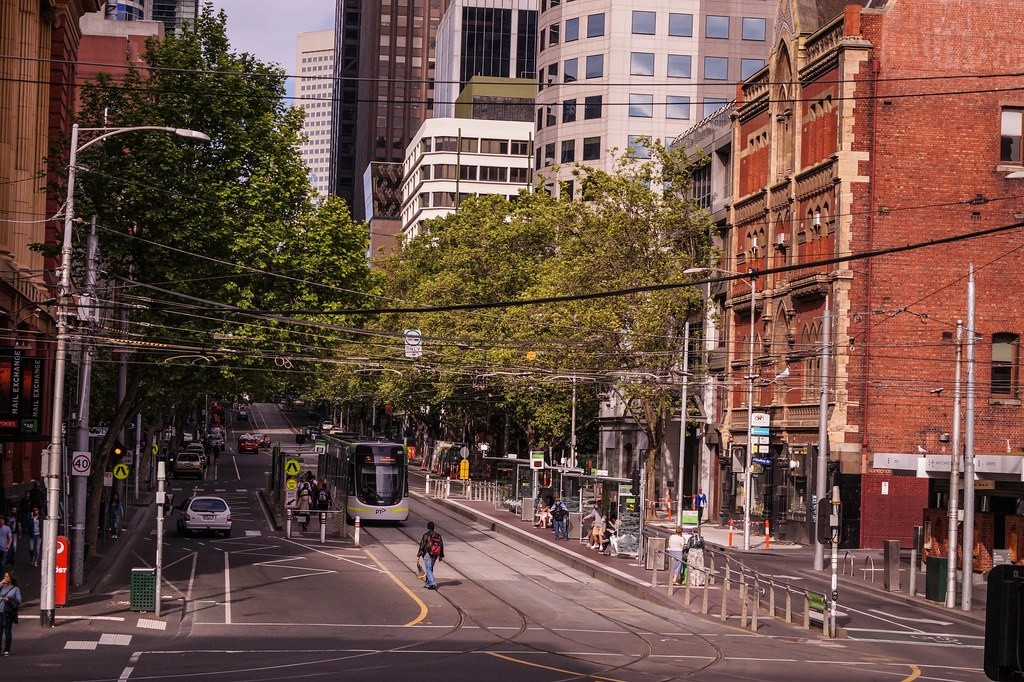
[[427, 521, 434, 529]]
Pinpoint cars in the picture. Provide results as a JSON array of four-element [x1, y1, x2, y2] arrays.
[[305, 420, 343, 441], [174, 408, 272, 476], [160, 427, 193, 442]]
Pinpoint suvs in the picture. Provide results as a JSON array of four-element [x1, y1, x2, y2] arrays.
[[174, 496, 233, 535]]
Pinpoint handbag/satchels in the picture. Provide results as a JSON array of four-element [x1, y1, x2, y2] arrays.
[[417, 564, 427, 579]]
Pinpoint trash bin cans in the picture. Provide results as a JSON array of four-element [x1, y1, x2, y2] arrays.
[[130, 568, 155, 612]]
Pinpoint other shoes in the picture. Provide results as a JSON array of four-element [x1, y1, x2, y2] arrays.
[[555, 538, 559, 540], [591, 544, 599, 549], [424, 584, 429, 588], [115, 535, 118, 538], [536, 522, 540, 525], [31, 562, 34, 564], [564, 538, 568, 541], [541, 526, 546, 529], [428, 584, 436, 590], [673, 581, 679, 586], [111, 535, 115, 538], [35, 564, 39, 567], [599, 545, 604, 551]]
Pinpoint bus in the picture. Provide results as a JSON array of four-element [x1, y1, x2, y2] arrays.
[[317, 432, 409, 523]]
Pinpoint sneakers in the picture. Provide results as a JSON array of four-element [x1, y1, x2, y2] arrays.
[[7, 561, 11, 565], [12, 563, 16, 566], [4, 651, 10, 656]]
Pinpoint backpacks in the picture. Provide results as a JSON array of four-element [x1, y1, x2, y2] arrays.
[[318, 489, 327, 501], [554, 502, 563, 521], [427, 532, 442, 555]]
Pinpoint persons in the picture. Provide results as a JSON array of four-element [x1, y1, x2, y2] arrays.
[[669, 526, 705, 585], [204, 441, 221, 467], [0, 481, 124, 579], [298, 470, 332, 535], [417, 521, 444, 590], [582, 500, 617, 556], [694, 488, 707, 525], [536, 497, 569, 541], [0, 572, 22, 657]]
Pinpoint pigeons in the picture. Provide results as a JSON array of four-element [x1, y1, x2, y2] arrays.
[[918, 445, 926, 453], [776, 368, 790, 379]]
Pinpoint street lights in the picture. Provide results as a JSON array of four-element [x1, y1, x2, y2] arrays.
[[679, 268, 755, 551], [39, 122, 213, 628]]
